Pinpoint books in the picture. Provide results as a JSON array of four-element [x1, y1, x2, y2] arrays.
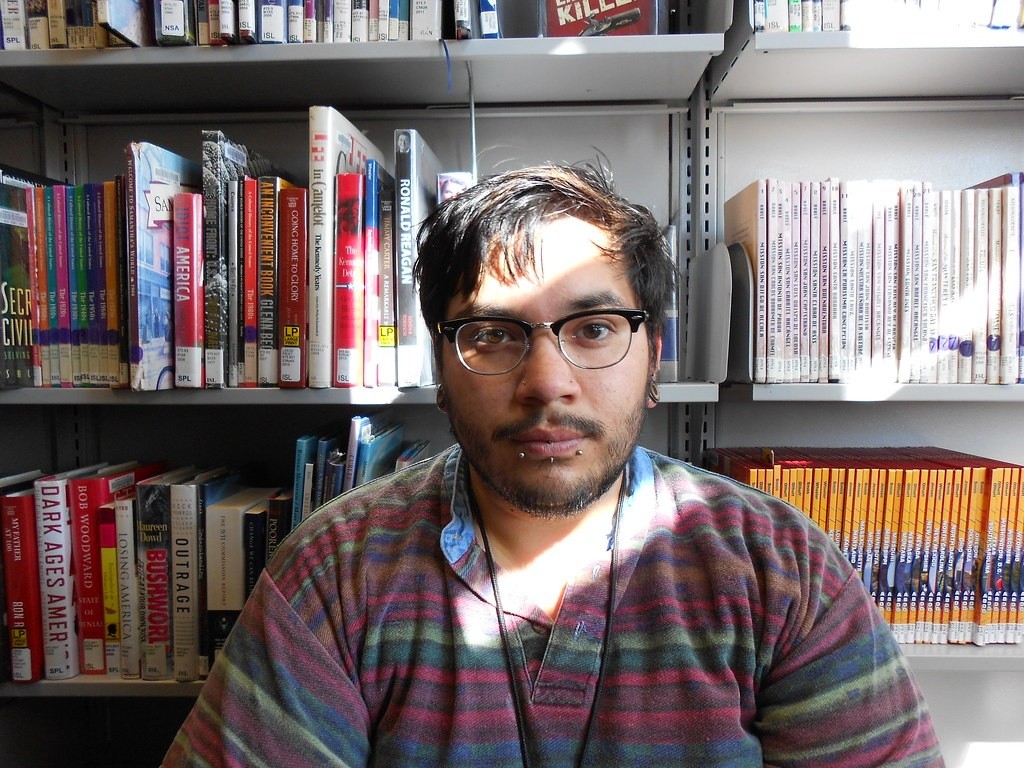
[[0, 106, 476, 392], [751, 0, 1024, 34], [724, 170, 1024, 386], [0, 0, 659, 51], [698, 446, 1024, 648], [0, 404, 431, 684]]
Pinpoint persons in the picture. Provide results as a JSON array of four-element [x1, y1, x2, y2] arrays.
[[396, 131, 411, 153], [159, 166, 944, 768], [440, 178, 468, 201]]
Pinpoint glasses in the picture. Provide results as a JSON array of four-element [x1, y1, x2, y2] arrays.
[[437, 309, 652, 375]]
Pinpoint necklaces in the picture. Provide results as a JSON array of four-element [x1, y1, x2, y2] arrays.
[[464, 457, 627, 768]]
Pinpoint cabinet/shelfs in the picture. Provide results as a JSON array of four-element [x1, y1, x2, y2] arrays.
[[1, 0, 1024, 768]]
